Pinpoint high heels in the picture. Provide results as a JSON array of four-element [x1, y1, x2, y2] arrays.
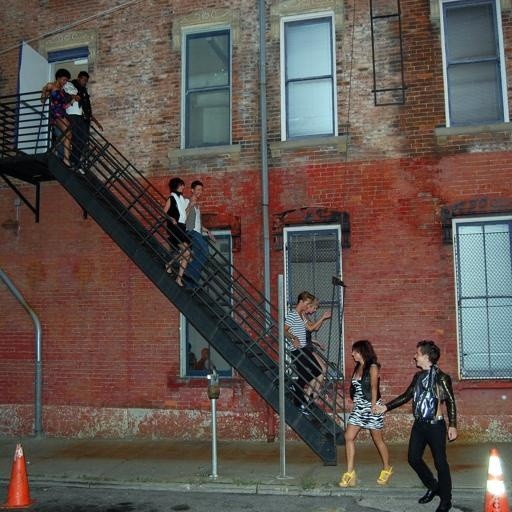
[[339, 470, 356, 487], [377, 469, 394, 485]]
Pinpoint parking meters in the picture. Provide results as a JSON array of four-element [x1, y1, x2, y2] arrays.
[[207, 371, 222, 479]]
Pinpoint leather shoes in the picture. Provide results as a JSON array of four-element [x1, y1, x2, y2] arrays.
[[436, 499, 453, 511], [418, 484, 438, 503]]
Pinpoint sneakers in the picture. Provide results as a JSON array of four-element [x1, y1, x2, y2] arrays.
[[299, 405, 310, 416]]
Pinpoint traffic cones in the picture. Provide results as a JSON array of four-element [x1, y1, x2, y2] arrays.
[[483, 448, 511, 512], [2, 443, 38, 511]]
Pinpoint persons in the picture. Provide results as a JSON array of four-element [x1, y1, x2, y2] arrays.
[[186, 180, 216, 287], [40, 68, 82, 168], [283, 291, 332, 415], [376, 340, 458, 511], [338, 339, 393, 488], [303, 298, 328, 407], [62, 71, 104, 176], [162, 176, 194, 288], [196, 347, 216, 369], [189, 343, 196, 370]]
